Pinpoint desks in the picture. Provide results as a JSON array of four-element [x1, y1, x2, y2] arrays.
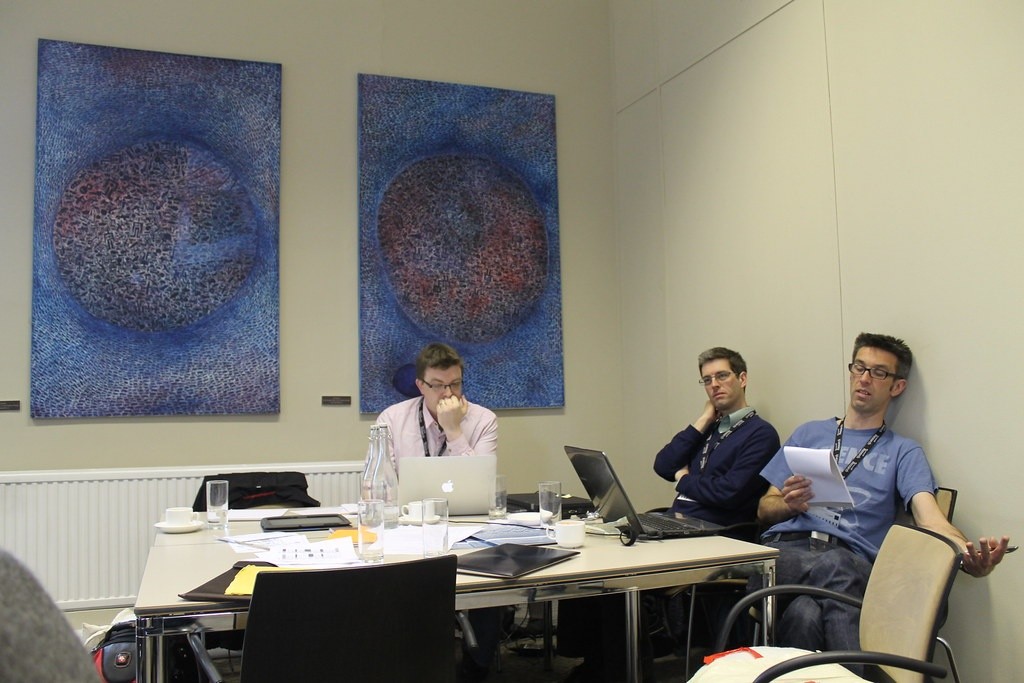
[[141, 536, 777, 683], [156, 510, 484, 544]]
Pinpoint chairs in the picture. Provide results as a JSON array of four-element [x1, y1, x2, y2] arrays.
[[682, 524, 965, 683], [193, 471, 312, 515], [886, 487, 958, 533], [185, 554, 459, 683]]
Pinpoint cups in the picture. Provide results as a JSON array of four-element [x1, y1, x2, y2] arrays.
[[545, 520, 586, 548], [166, 507, 200, 525], [538, 481, 562, 529], [422, 498, 450, 558], [358, 500, 384, 562], [206, 480, 229, 529], [489, 475, 507, 520], [401, 501, 435, 519]]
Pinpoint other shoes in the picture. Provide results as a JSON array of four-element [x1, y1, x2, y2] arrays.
[[461, 649, 489, 681]]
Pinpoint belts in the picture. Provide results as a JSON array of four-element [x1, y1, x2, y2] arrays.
[[769, 531, 849, 551]]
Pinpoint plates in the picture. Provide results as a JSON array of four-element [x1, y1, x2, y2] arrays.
[[399, 515, 440, 525], [154, 521, 204, 533]]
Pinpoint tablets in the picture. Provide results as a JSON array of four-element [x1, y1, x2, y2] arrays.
[[260, 514, 351, 529]]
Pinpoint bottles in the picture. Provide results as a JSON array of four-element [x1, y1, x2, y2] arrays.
[[361, 424, 379, 499], [373, 424, 400, 528]]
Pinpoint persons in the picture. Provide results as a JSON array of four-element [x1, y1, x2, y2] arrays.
[[376, 342, 503, 683], [553, 347, 781, 683], [752, 333, 1009, 676]]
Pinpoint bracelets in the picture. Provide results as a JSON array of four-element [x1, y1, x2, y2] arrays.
[[959, 559, 967, 575]]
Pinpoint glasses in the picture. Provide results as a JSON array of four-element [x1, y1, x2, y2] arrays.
[[699, 372, 739, 387], [422, 378, 461, 392], [848, 363, 903, 379]]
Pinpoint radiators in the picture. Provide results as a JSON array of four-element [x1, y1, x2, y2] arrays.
[[0, 459, 368, 615]]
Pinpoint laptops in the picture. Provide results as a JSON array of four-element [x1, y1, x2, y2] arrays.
[[505, 491, 596, 514], [397, 454, 497, 515], [564, 446, 726, 540]]
